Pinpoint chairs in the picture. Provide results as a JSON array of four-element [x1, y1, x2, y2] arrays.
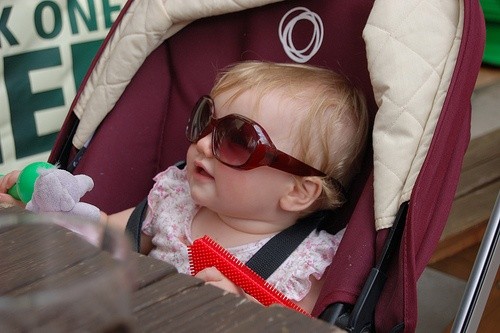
[[44, 0, 500, 333]]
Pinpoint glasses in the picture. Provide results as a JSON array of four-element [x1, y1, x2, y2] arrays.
[[186, 95, 348, 196]]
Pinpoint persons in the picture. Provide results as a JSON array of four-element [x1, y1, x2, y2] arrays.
[[2, 56, 370, 317]]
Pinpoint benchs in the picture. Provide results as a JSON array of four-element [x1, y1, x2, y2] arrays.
[[417, 128, 500, 280]]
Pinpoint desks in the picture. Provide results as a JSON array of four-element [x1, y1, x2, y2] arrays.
[[0, 203, 344, 333]]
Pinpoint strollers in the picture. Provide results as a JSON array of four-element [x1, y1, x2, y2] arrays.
[[1, 0, 500, 333]]
